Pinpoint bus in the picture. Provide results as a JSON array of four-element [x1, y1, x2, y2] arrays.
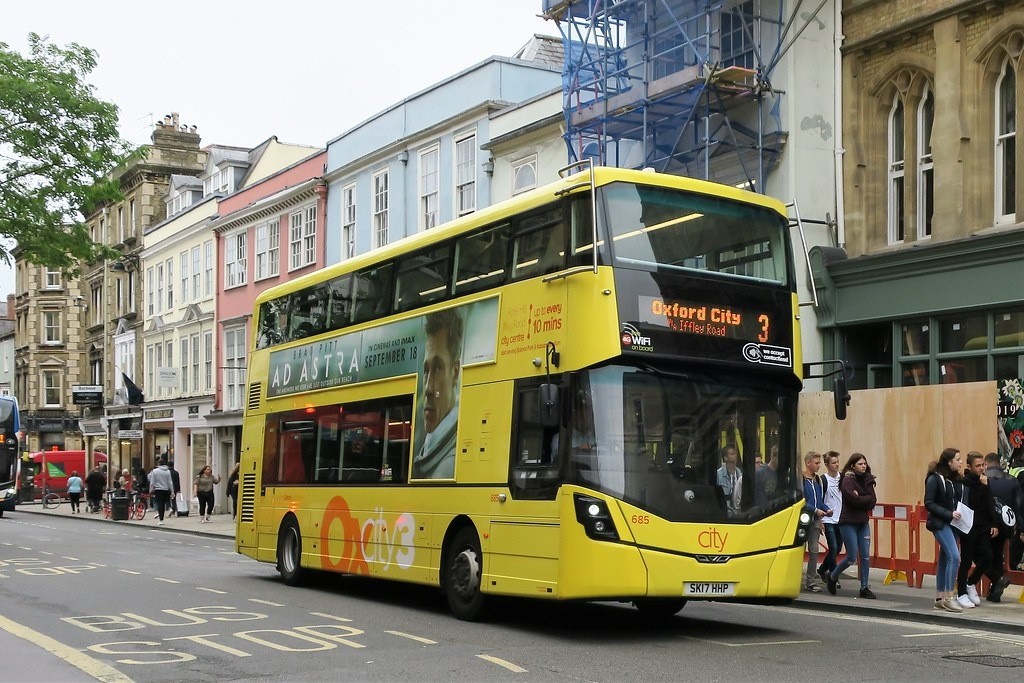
[[0, 394, 30, 521], [235, 155, 852, 622]]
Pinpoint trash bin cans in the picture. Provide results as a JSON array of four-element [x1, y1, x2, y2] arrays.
[[112, 498, 129, 521]]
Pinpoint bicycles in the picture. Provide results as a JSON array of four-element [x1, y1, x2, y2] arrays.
[[103, 487, 147, 520], [38, 479, 60, 508]]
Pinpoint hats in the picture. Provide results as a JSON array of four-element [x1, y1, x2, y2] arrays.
[[156, 459, 164, 463], [166, 461, 173, 465]]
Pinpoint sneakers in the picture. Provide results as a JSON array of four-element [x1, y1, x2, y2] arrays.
[[956, 593, 976, 608], [967, 584, 980, 606], [942, 597, 963, 611], [933, 598, 945, 609]]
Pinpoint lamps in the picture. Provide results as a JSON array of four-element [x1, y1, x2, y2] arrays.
[[110, 254, 140, 280]]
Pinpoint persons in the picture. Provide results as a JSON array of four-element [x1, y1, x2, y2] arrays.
[[924, 447, 1024, 613], [84, 465, 106, 514], [225, 462, 240, 520], [65, 470, 83, 514], [717, 443, 878, 600], [413, 308, 464, 478], [550, 393, 608, 465], [113, 453, 180, 526], [193, 465, 221, 524]]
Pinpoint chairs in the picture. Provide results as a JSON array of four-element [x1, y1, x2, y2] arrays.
[[258, 289, 424, 348]]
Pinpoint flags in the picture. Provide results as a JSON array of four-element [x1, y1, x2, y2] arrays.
[[123, 373, 144, 407]]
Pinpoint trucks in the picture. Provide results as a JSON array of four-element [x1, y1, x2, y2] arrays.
[[19, 451, 107, 499]]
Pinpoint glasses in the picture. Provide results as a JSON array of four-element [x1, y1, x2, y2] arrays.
[[755, 461, 763, 466]]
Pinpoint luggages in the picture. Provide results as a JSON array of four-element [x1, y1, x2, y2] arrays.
[[175, 492, 190, 518]]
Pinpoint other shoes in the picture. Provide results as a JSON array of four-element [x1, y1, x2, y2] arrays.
[[816, 569, 826, 583], [91, 506, 95, 514], [159, 520, 164, 525], [201, 518, 205, 523], [826, 572, 837, 595], [805, 581, 822, 592], [154, 514, 159, 518], [169, 510, 173, 517], [836, 582, 842, 589], [859, 588, 876, 598], [987, 576, 1010, 602], [206, 517, 211, 523], [94, 511, 98, 514], [77, 507, 80, 513]]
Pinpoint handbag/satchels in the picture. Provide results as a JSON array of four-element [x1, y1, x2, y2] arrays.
[[992, 496, 1017, 538]]
